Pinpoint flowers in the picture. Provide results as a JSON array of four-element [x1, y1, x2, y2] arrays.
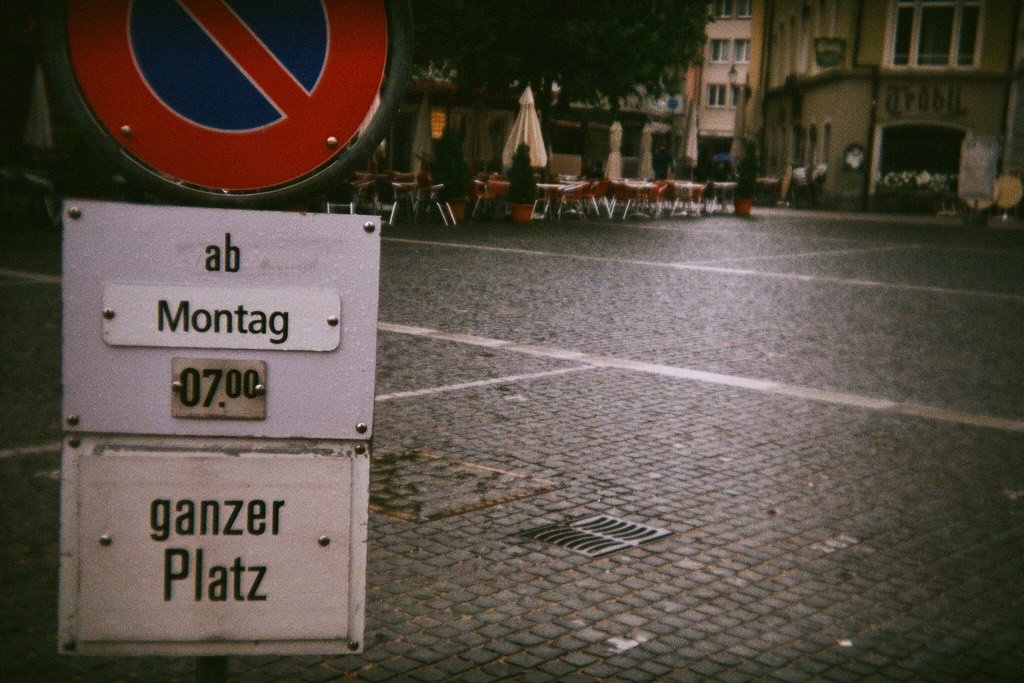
[[877, 168, 946, 192]]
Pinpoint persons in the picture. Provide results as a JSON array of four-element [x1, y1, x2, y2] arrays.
[[652, 139, 675, 181], [874, 163, 969, 218], [504, 144, 536, 207], [332, 127, 475, 221], [691, 143, 871, 217]]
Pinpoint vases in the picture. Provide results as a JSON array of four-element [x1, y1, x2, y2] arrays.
[[874, 188, 943, 216]]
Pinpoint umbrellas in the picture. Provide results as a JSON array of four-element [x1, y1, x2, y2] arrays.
[[676, 95, 699, 184], [502, 85, 548, 178]]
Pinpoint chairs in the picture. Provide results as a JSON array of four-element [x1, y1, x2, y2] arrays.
[[323, 160, 774, 232]]
